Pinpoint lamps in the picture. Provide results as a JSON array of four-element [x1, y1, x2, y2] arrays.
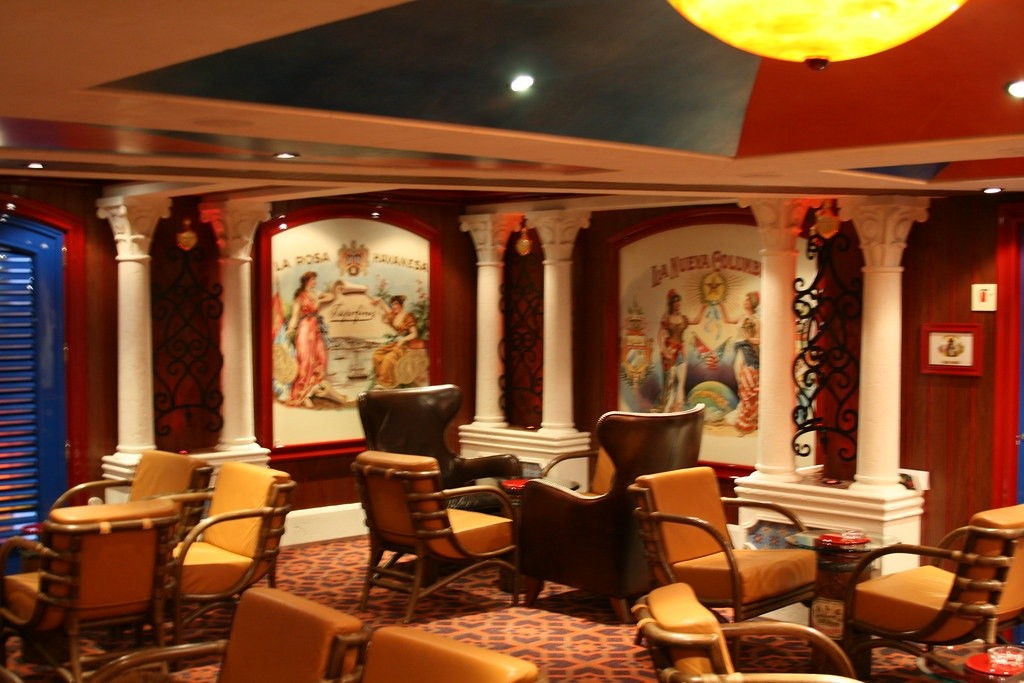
[[666, 0, 968, 70]]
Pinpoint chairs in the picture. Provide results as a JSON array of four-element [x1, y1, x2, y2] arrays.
[[49, 449, 213, 646], [520, 402, 706, 622], [159, 460, 297, 671], [0, 496, 181, 683], [352, 450, 518, 624], [627, 465, 817, 645], [842, 502, 1024, 683], [357, 384, 523, 589], [85, 587, 368, 683], [324, 624, 549, 683], [629, 579, 864, 683]]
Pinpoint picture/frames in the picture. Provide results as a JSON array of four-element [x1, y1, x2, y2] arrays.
[[919, 322, 985, 375]]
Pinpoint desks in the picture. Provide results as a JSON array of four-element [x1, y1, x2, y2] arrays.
[[16, 522, 52, 575], [785, 529, 903, 682], [498, 478, 580, 593], [916, 643, 1024, 683]]
